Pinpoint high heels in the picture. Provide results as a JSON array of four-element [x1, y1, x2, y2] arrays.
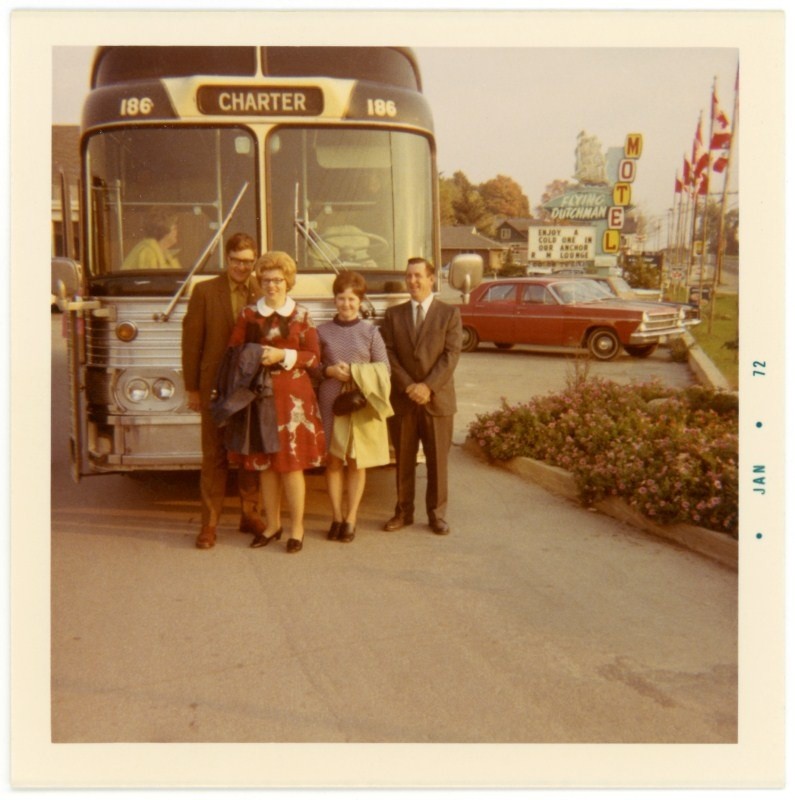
[[287, 533, 304, 553], [250, 526, 283, 548]]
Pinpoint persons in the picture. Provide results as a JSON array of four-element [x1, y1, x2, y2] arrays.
[[119, 208, 182, 270], [382, 257, 463, 536], [306, 271, 394, 544], [227, 250, 326, 554], [180, 233, 267, 549]]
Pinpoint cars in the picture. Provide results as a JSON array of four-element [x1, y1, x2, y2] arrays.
[[450, 277, 703, 363], [576, 274, 639, 300]]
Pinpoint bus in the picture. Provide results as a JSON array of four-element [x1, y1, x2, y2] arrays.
[[51, 47, 484, 490], [51, 123, 86, 305]]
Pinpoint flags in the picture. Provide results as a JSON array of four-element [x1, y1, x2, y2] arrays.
[[673, 63, 738, 198]]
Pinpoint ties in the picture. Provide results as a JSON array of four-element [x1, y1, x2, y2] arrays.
[[236, 284, 246, 317], [416, 305, 423, 337]]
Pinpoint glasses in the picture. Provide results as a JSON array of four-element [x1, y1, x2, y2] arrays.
[[262, 278, 284, 284], [228, 256, 255, 266]]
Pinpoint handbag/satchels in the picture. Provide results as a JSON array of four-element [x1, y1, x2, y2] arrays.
[[333, 366, 367, 416]]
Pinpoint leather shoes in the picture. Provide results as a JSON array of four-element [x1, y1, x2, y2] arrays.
[[384, 514, 414, 531], [429, 518, 449, 534]]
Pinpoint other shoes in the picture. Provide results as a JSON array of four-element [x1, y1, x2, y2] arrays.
[[240, 520, 265, 536], [328, 519, 342, 540], [196, 529, 216, 548], [341, 520, 356, 542]]
